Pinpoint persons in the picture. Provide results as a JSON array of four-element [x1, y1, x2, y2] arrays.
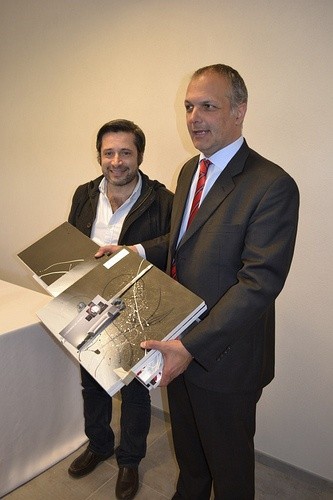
[[68, 119, 175, 500], [94, 64, 300, 500]]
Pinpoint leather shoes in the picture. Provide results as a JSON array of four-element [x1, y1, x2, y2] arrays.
[[115, 463, 139, 500], [68, 447, 115, 478]]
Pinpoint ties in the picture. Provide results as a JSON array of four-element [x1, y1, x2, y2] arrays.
[[171, 159, 213, 281]]
